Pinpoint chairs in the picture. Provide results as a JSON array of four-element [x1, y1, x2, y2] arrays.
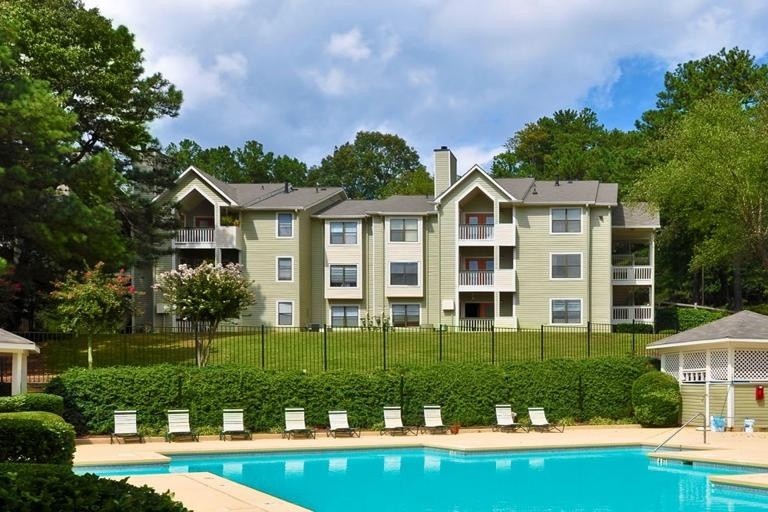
[[494, 401, 565, 435]]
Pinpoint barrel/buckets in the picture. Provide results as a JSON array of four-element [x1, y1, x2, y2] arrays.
[[744, 418, 754, 434]]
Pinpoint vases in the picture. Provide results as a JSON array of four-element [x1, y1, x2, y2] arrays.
[[450, 422, 462, 434]]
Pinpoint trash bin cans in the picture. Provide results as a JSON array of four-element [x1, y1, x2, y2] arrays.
[[439, 324, 448, 332], [744, 419, 755, 432], [312, 324, 319, 332]]
